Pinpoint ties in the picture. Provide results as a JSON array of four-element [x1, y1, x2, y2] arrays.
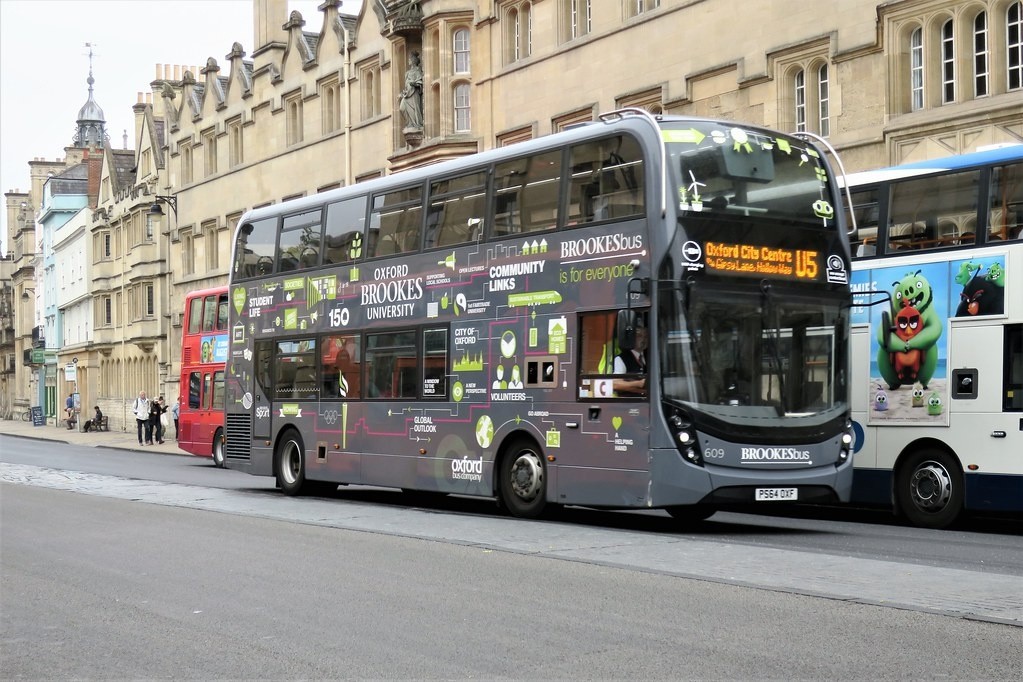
[[638, 354, 644, 366]]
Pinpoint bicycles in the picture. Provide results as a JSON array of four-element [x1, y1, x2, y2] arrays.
[[21, 408, 32, 422]]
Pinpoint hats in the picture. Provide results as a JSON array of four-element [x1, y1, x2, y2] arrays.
[[154, 397, 159, 401]]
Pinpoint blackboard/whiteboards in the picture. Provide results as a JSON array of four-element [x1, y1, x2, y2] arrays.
[[31, 407, 43, 426]]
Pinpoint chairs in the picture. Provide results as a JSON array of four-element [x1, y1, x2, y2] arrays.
[[91, 416, 108, 432], [242, 248, 318, 277]]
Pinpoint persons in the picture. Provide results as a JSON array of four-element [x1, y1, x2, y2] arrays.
[[62, 394, 102, 432], [396, 50, 425, 128], [613, 322, 648, 397], [296, 348, 360, 399], [133, 391, 180, 446]]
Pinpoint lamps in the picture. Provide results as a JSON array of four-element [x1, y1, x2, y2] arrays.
[[145, 195, 177, 221]]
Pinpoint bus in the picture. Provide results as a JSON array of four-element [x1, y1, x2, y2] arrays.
[[176, 106, 862, 519], [662, 143, 1022, 530]]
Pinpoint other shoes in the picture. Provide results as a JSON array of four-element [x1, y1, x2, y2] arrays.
[[139, 442, 149, 446], [148, 440, 153, 445], [159, 441, 164, 444]]
